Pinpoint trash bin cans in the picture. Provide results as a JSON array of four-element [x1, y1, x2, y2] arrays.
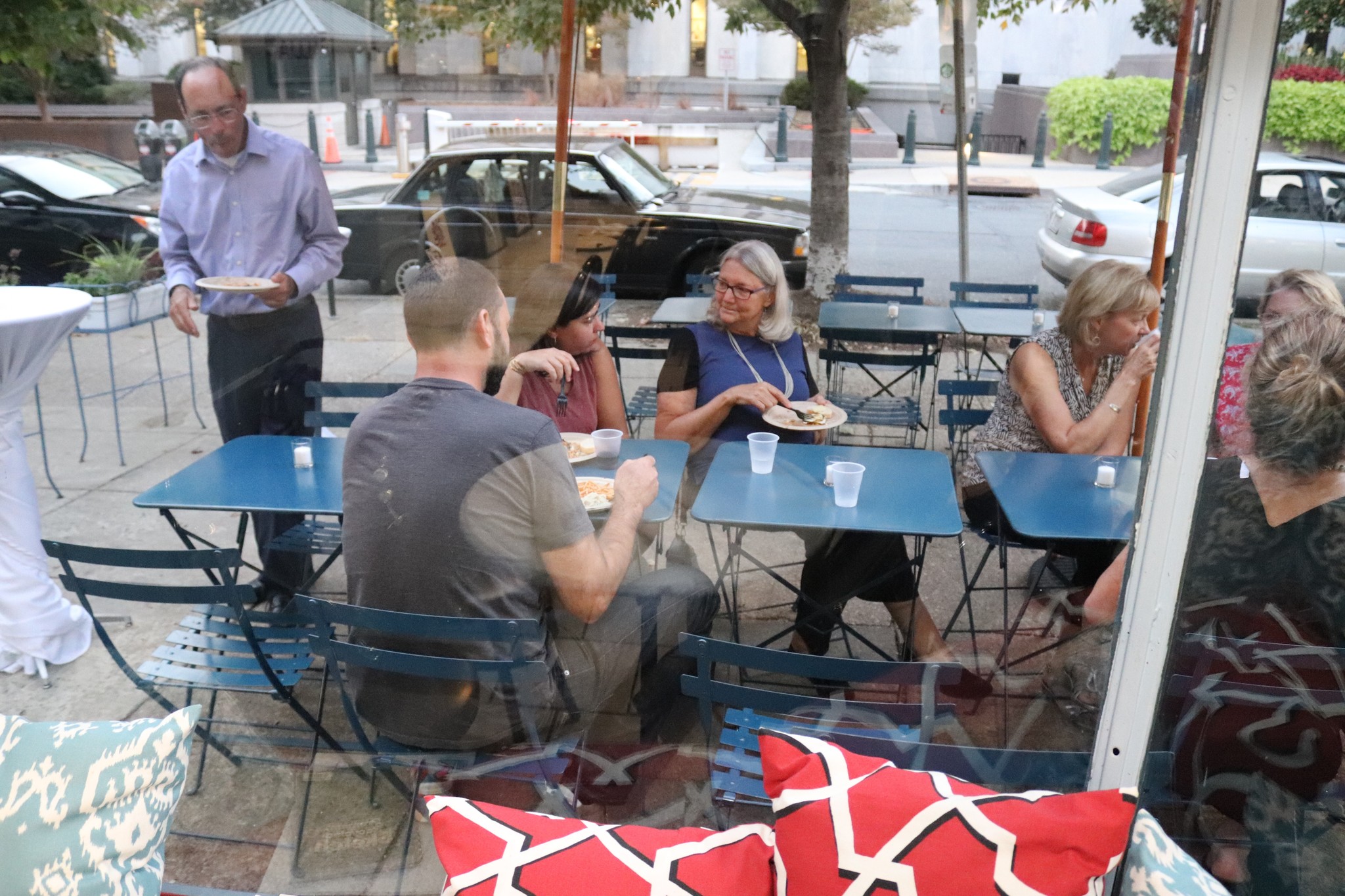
[[160, 119, 189, 166], [135, 121, 162, 182]]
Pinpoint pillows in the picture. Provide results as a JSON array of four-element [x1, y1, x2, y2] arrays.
[[758, 726, 1139, 896], [422, 795, 774, 896], [0, 704, 203, 896]]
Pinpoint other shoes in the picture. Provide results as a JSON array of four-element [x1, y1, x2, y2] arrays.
[[909, 651, 993, 700], [1201, 846, 1254, 896], [270, 596, 296, 627], [787, 642, 855, 702], [247, 576, 270, 603]]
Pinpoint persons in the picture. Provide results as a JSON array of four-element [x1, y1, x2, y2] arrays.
[[157, 55, 348, 626], [491, 263, 631, 440], [962, 260, 1160, 645], [1084, 269, 1345, 896], [341, 256, 722, 753], [654, 240, 994, 701]]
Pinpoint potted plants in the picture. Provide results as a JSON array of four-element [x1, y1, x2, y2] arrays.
[[62, 224, 170, 329]]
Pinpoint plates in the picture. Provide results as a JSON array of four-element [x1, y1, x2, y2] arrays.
[[195, 277, 281, 293], [762, 401, 848, 431], [575, 476, 615, 513], [560, 432, 596, 463]]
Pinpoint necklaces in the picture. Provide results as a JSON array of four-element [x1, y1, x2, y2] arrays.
[[728, 328, 794, 402]]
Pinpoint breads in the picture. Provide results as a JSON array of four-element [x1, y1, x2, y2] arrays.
[[806, 405, 833, 418], [579, 439, 595, 455]]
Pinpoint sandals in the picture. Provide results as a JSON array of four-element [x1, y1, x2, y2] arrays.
[[1041, 653, 1100, 729]]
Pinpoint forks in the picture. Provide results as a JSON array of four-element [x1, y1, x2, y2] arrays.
[[556, 372, 568, 417], [777, 401, 816, 420]]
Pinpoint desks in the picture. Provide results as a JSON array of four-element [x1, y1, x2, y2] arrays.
[[571, 439, 690, 575], [953, 307, 1062, 459], [133, 434, 373, 786], [817, 302, 962, 447], [689, 441, 963, 702], [506, 297, 616, 315], [646, 297, 711, 328], [0, 287, 93, 683], [941, 451, 1142, 716]]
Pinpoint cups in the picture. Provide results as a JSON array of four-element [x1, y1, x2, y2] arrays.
[[887, 301, 900, 318], [591, 428, 623, 470], [1135, 328, 1161, 353], [291, 437, 314, 468], [747, 432, 780, 474], [1033, 308, 1046, 326], [831, 462, 866, 507], [823, 455, 846, 487], [1094, 455, 1120, 488]]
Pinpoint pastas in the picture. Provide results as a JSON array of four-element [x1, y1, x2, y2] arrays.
[[577, 481, 614, 501]]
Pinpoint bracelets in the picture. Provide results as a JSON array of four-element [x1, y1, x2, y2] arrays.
[[509, 358, 527, 375], [1101, 397, 1121, 414]]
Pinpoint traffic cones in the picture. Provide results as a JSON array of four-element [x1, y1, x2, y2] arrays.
[[322, 115, 342, 163], [377, 115, 392, 149]]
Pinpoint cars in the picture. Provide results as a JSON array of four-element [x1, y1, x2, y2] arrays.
[[1036, 151, 1345, 318], [333, 135, 812, 298], [0, 140, 163, 284]]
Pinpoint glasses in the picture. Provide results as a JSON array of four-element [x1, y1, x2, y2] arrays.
[[181, 94, 239, 130], [711, 274, 770, 300], [1257, 312, 1279, 324]]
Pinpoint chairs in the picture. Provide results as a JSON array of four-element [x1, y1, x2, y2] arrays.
[[1274, 183, 1309, 220], [455, 178, 480, 204], [34, 275, 1345, 896]]
